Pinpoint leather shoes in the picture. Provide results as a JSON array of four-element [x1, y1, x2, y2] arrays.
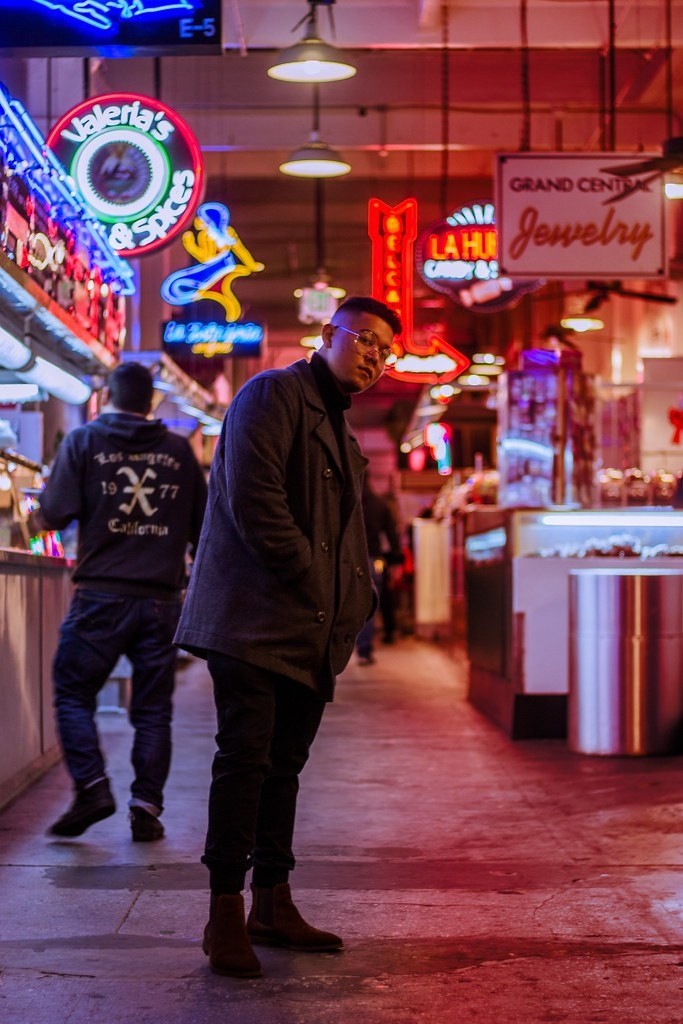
[[198, 893, 265, 980], [245, 882, 345, 950]]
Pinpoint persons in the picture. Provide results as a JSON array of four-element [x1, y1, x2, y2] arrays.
[[172, 296, 403, 978], [26, 360, 208, 849], [358, 465, 432, 666]]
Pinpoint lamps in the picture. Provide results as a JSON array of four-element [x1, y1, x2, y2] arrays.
[[264, 0, 360, 87], [278, 82, 351, 180]]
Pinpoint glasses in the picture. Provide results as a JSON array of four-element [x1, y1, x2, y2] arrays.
[[335, 324, 398, 375]]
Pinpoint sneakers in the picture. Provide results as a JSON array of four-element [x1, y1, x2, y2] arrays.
[[128, 805, 165, 843], [49, 778, 117, 839]]
